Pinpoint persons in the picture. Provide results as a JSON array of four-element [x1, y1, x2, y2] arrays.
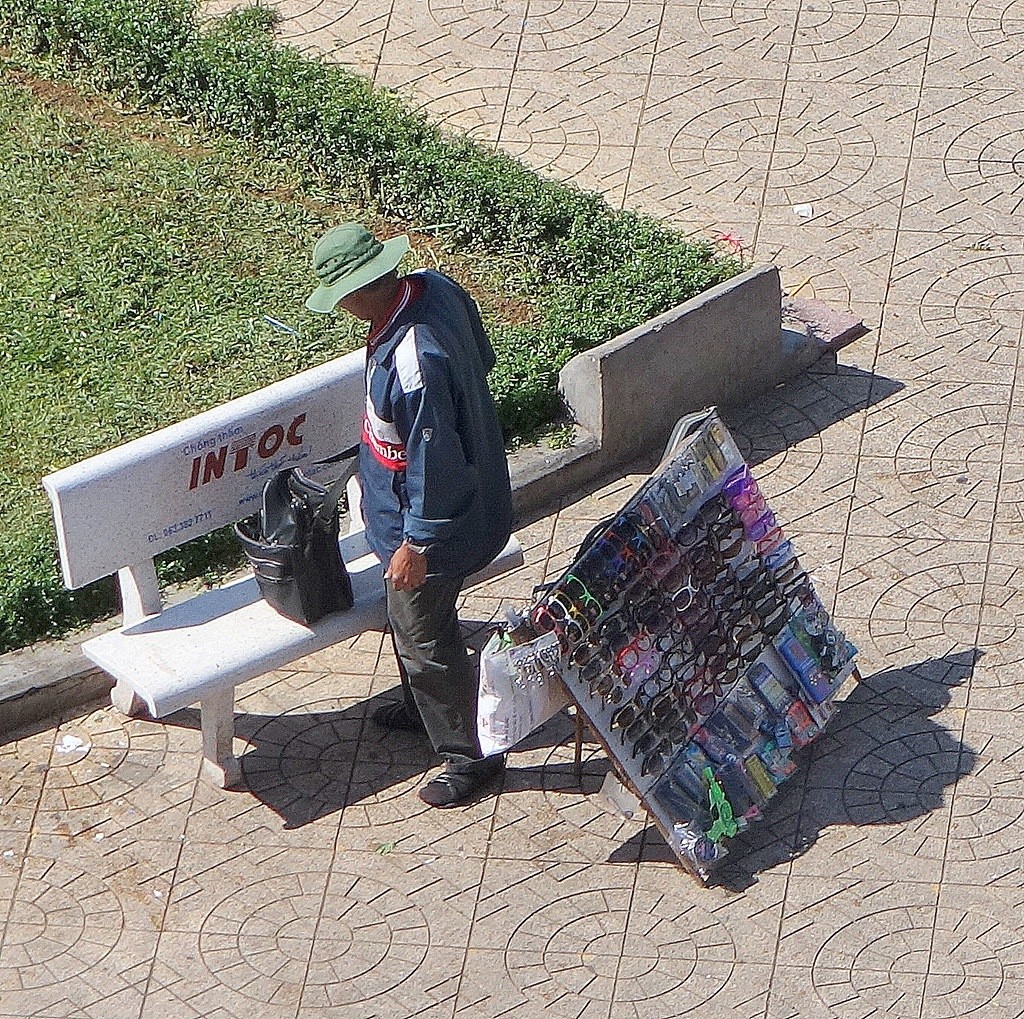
[[304, 221, 512, 807]]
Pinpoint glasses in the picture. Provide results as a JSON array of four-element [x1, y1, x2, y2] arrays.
[[534, 464, 814, 778]]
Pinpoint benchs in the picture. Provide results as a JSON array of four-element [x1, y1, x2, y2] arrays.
[[43, 347, 522, 791]]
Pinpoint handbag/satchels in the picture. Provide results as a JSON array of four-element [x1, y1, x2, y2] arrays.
[[235, 444, 361, 625]]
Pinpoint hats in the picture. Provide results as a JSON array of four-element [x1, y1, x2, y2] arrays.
[[305, 222, 410, 313]]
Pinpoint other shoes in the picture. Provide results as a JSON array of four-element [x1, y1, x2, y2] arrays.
[[373, 700, 422, 732], [420, 759, 504, 808]]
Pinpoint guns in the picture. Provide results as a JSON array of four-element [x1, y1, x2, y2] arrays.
[[703, 766, 737, 842]]
